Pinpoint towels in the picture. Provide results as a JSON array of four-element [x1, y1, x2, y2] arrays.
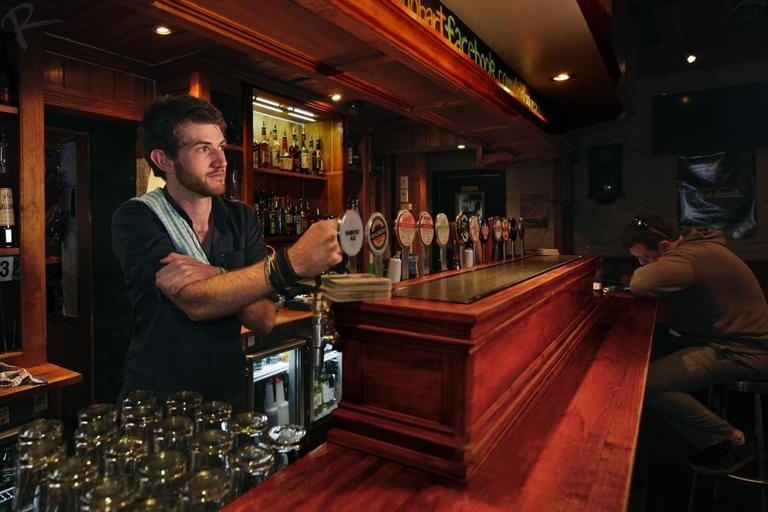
[[0, 364, 48, 387], [123, 187, 212, 270]]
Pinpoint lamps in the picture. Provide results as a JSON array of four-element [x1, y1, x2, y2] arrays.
[[253, 96, 319, 123], [458, 145, 466, 149], [156, 26, 171, 35], [332, 94, 341, 102], [552, 73, 571, 84]]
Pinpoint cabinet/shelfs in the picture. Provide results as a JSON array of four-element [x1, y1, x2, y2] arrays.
[[152, 74, 245, 210], [249, 83, 343, 252], [343, 105, 397, 283], [325, 247, 601, 480], [0, 21, 24, 362]]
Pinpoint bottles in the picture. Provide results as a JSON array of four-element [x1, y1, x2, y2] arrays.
[[270, 124, 280, 171], [263, 381, 279, 430], [313, 133, 324, 176], [351, 198, 355, 211], [275, 378, 290, 426], [347, 145, 353, 164], [321, 360, 336, 410], [309, 134, 315, 173], [0, 146, 16, 249], [280, 130, 293, 171], [300, 125, 310, 174], [254, 184, 321, 238], [354, 194, 360, 212], [288, 127, 300, 172], [257, 120, 273, 168]]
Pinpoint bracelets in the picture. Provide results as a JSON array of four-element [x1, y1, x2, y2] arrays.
[[277, 247, 302, 283]]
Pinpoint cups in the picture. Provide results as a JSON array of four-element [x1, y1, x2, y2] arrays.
[[226, 442, 276, 496], [121, 389, 159, 415], [77, 403, 117, 426], [262, 423, 307, 467], [228, 412, 268, 440], [153, 416, 194, 451], [190, 429, 235, 466], [195, 401, 232, 428], [121, 405, 164, 439], [124, 495, 181, 512], [1, 420, 63, 481], [74, 422, 116, 461], [103, 436, 150, 475], [78, 477, 133, 512], [178, 467, 233, 511], [13, 442, 65, 511], [166, 387, 203, 415], [34, 463, 96, 511], [137, 452, 187, 493]]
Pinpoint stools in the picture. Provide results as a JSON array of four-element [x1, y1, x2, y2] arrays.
[[687, 380, 767, 511]]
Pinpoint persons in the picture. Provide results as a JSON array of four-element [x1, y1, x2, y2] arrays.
[[111, 95, 343, 410], [623, 214, 768, 473]]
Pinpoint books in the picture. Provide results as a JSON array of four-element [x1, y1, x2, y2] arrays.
[[322, 273, 391, 303]]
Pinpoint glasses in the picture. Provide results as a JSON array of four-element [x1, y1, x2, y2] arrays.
[[630, 216, 667, 244]]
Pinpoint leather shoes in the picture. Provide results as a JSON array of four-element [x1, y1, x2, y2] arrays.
[[691, 439, 757, 475]]
